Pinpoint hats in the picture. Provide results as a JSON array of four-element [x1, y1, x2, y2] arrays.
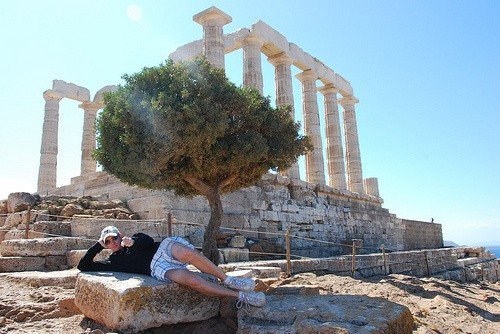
[[101, 225, 120, 243]]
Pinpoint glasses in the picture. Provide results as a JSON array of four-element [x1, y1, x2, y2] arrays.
[[104, 237, 118, 244]]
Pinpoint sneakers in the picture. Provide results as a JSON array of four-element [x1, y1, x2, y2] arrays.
[[228, 277, 255, 291], [242, 292, 266, 307]]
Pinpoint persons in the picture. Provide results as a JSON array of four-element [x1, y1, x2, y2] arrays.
[[77, 225, 266, 312]]
